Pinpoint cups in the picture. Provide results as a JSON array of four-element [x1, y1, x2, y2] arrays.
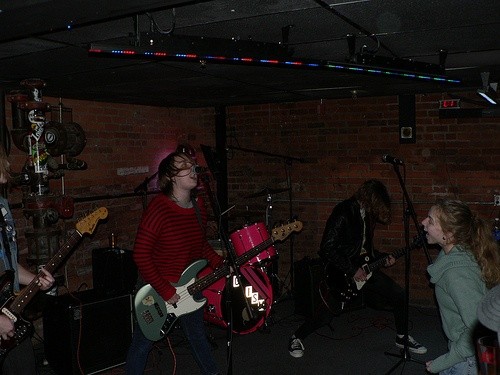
[[476, 335, 499, 375], [108, 232, 119, 249]]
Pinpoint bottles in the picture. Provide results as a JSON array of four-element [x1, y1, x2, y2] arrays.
[[493, 218, 500, 244]]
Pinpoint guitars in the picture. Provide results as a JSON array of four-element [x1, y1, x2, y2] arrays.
[[133, 217, 303, 345], [319, 236, 428, 311], [195, 260, 274, 336], [0, 205, 108, 361]]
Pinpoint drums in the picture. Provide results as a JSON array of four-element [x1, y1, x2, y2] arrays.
[[228, 221, 279, 268]]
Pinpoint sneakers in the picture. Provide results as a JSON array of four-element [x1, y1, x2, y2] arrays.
[[395, 334, 427, 354], [288, 333, 304, 357]]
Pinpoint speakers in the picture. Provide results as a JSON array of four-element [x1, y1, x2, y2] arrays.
[[41, 286, 133, 374], [295, 259, 366, 318]]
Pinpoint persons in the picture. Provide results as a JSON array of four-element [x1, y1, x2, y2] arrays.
[[124, 151, 239, 375], [0, 143, 55, 375], [420, 199, 490, 375], [289, 178, 428, 358]]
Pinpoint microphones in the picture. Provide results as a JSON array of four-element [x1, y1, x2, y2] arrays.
[[191, 165, 206, 174], [382, 154, 404, 165]]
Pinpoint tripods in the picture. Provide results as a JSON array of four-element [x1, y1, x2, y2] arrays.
[[384, 164, 434, 375], [228, 146, 306, 304]]
